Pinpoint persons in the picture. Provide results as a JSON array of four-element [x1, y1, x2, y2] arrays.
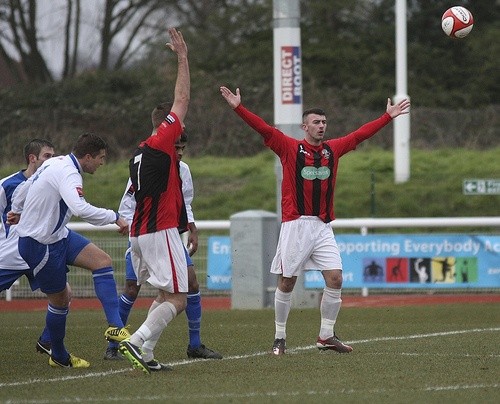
[[6, 133, 132, 369], [220, 86, 411, 354], [0, 140, 71, 356], [104, 136, 223, 359], [117, 27, 191, 376]]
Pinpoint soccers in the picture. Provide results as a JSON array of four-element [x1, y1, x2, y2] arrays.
[[441, 6, 473, 40]]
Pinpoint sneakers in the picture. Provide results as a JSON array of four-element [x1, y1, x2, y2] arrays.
[[48, 353, 90, 368], [271, 338, 286, 355], [145, 359, 172, 371], [118, 340, 151, 375], [36, 339, 53, 356], [104, 347, 125, 361], [187, 344, 223, 359], [317, 332, 353, 354], [104, 325, 132, 343]]
